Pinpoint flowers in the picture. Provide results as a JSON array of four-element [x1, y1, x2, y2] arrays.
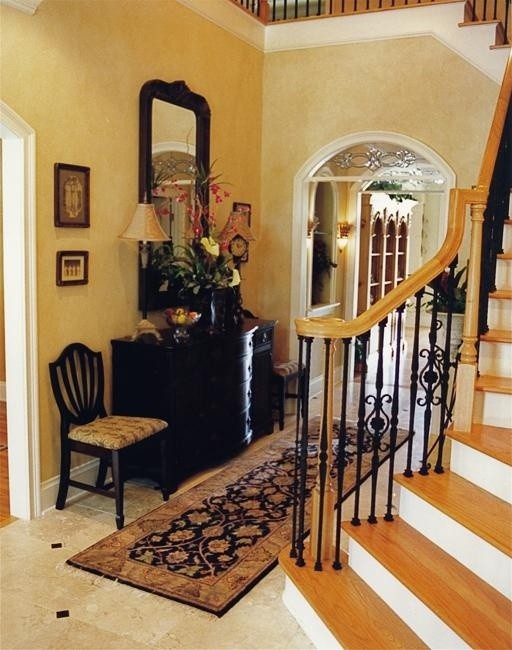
[[148, 154, 189, 221], [410, 265, 470, 311], [157, 235, 243, 292], [174, 150, 250, 292]]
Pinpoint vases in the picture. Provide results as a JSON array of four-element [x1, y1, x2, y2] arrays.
[[436, 311, 465, 363], [178, 284, 218, 338], [210, 286, 245, 336]]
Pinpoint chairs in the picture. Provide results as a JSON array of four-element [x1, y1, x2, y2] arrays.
[[45, 342, 172, 531], [240, 306, 308, 432]]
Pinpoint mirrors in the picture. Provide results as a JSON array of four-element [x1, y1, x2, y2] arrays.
[[138, 77, 211, 309]]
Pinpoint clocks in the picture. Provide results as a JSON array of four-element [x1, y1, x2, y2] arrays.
[[227, 233, 258, 320]]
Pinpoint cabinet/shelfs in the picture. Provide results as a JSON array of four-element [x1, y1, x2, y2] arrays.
[[108, 317, 279, 497], [357, 191, 419, 365]]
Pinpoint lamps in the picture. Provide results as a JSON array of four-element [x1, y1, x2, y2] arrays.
[[336, 222, 353, 252], [216, 211, 260, 333], [304, 219, 320, 250], [119, 190, 173, 342]]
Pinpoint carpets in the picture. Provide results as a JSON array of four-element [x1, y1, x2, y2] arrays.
[[65, 410, 414, 617]]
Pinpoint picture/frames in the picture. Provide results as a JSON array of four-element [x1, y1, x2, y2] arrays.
[[232, 201, 252, 229], [56, 249, 89, 287], [53, 161, 91, 228]]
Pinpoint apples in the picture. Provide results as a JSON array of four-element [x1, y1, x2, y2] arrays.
[[165, 308, 197, 325]]
